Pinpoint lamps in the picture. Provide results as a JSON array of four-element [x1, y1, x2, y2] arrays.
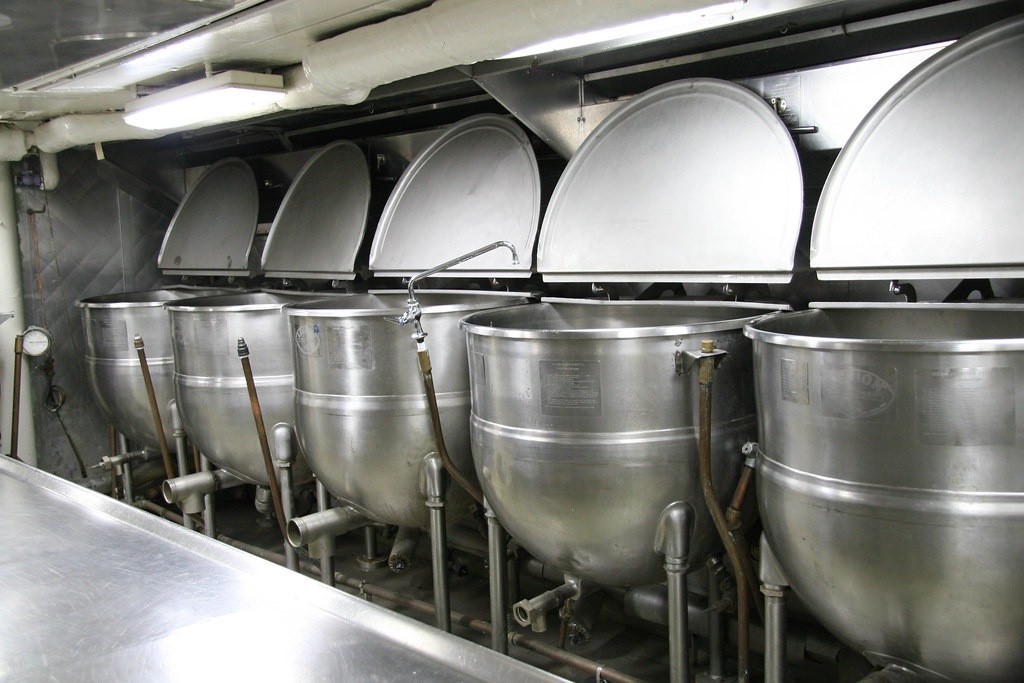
[[122, 63, 286, 131]]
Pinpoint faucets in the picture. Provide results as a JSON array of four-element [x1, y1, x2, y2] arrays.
[[382, 240, 519, 328]]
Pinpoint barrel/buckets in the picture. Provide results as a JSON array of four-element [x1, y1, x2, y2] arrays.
[[745, 308, 1024, 683], [73, 289, 252, 452], [164, 292, 362, 484], [458, 305, 782, 588], [279, 293, 528, 528]]
